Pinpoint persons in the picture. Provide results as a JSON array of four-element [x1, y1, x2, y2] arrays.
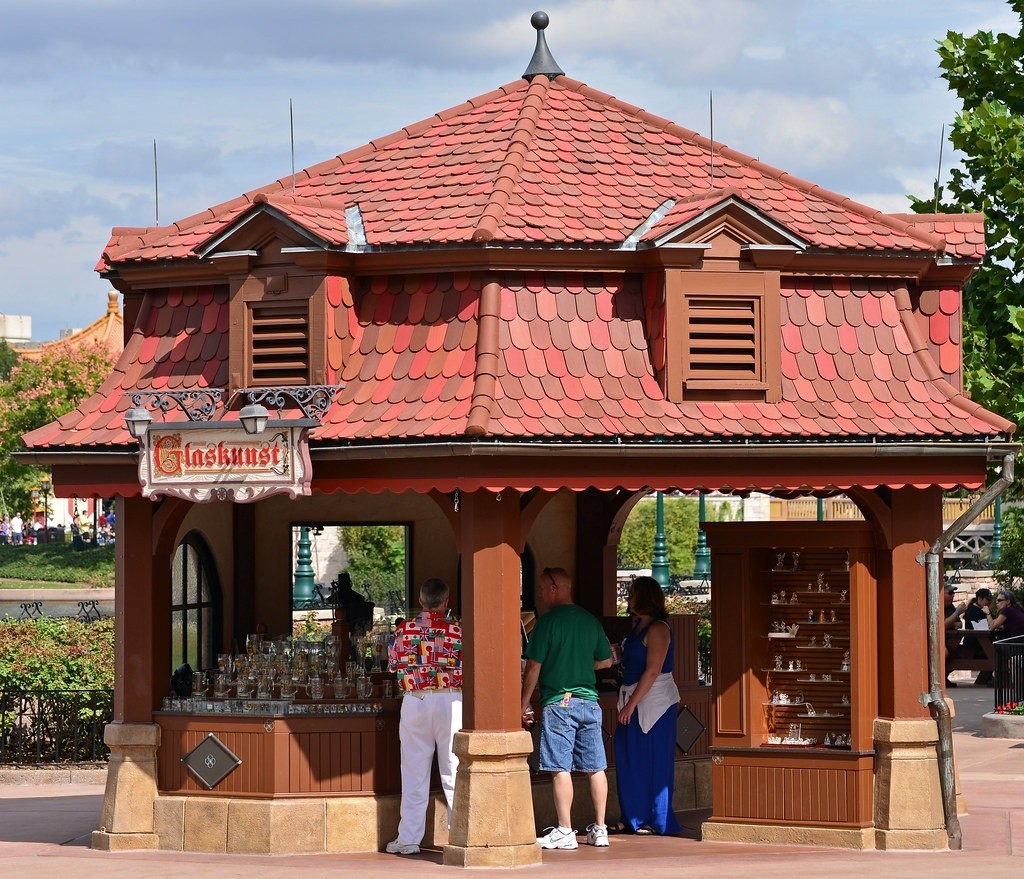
[[518, 566, 614, 850], [385, 577, 464, 855], [981, 590, 1024, 688], [963, 588, 996, 687], [612, 576, 681, 835], [325, 572, 366, 676], [0, 502, 116, 546], [944, 582, 967, 688]]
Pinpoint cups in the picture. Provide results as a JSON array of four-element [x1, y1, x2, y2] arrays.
[[236, 674, 255, 700], [371, 634, 382, 672], [217, 634, 340, 675], [281, 675, 298, 698], [351, 634, 367, 673], [357, 678, 374, 699], [332, 677, 352, 700], [257, 675, 274, 698], [607, 643, 621, 666], [213, 673, 232, 699], [190, 672, 209, 700], [305, 677, 326, 700], [346, 661, 356, 676]]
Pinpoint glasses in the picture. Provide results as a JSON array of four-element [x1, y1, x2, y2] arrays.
[[944, 591, 954, 595], [997, 599, 1007, 602], [543, 567, 557, 586]]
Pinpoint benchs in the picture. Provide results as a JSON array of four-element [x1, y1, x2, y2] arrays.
[[946, 629, 1012, 671]]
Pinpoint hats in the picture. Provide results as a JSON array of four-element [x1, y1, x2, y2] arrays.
[[944, 582, 958, 591]]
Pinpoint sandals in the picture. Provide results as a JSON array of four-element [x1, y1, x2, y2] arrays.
[[635, 827, 656, 835], [607, 823, 628, 833]]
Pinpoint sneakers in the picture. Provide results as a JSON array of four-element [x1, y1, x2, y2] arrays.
[[536, 827, 578, 849], [586, 823, 609, 846], [386, 839, 420, 854]]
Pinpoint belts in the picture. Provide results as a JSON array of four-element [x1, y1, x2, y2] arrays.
[[405, 687, 461, 700]]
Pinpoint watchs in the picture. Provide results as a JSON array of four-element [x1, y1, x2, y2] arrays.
[[986, 612, 991, 616]]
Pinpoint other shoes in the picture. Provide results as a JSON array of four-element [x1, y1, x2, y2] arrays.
[[946, 679, 957, 688]]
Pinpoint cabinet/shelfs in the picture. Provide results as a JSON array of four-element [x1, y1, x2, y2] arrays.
[[699, 521, 878, 830]]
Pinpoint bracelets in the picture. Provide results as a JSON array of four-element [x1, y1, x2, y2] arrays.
[[954, 610, 960, 615]]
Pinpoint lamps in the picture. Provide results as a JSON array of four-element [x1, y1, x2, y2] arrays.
[[118, 377, 229, 438], [232, 376, 345, 435]]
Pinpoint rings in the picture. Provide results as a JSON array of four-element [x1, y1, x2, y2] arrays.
[[624, 719, 627, 720]]
